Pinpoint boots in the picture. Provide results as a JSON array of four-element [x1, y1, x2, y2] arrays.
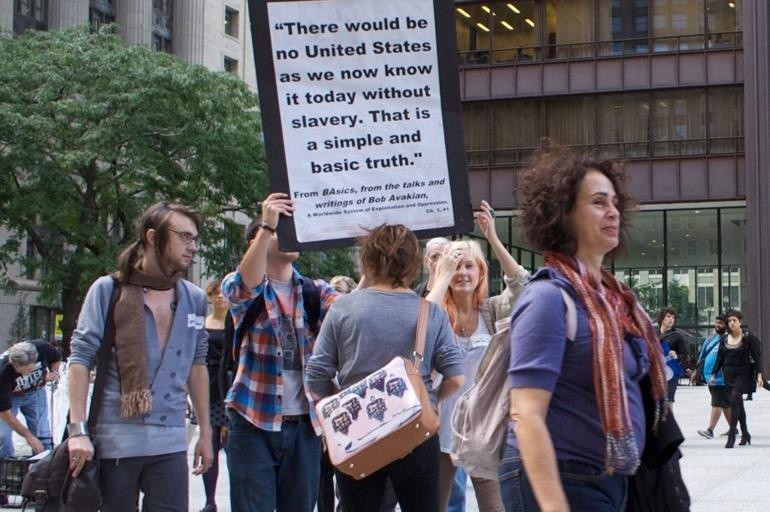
[[725, 400, 739, 448], [736, 399, 750, 445]]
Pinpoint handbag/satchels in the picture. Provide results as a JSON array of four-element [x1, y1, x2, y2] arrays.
[[450, 285, 577, 480], [20, 429, 102, 512], [315, 355, 440, 481], [692, 357, 707, 386]]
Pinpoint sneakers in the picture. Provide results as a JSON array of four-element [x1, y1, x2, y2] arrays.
[[698, 430, 713, 439]]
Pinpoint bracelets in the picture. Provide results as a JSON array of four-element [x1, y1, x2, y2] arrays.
[[66, 421, 91, 438]]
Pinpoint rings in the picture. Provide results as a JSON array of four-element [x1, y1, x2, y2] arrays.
[[72, 456, 80, 463]]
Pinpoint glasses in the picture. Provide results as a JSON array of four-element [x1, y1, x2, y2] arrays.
[[166, 229, 202, 247]]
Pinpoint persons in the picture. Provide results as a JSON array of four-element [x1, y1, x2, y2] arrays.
[[655, 306, 764, 448], [202, 192, 531, 512], [67, 201, 215, 512], [0, 338, 61, 508], [499, 145, 690, 512]]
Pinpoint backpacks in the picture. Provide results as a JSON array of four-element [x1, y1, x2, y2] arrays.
[[217, 278, 321, 400]]
